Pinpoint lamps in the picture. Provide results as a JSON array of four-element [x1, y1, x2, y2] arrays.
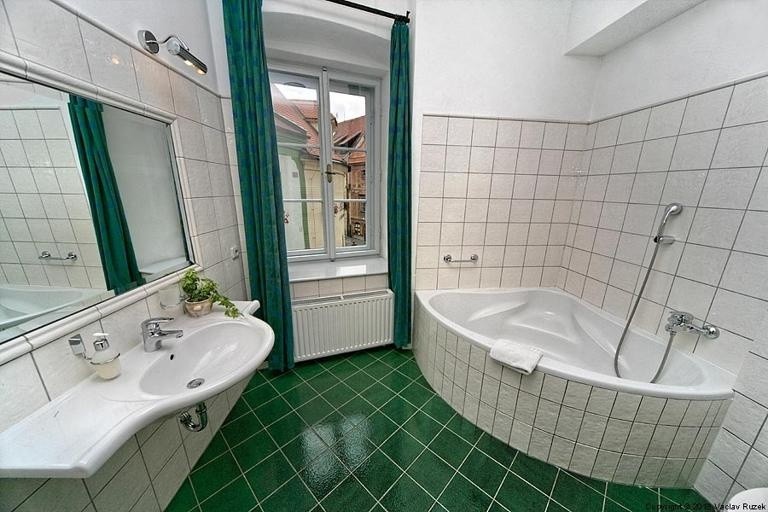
[[137, 29, 209, 77]]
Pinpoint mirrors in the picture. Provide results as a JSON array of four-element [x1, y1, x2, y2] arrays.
[[0, 48, 204, 365]]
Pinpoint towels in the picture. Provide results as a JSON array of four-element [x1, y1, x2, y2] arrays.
[[490, 338, 544, 375]]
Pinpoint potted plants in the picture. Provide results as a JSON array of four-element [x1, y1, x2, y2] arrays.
[[182, 268, 244, 319]]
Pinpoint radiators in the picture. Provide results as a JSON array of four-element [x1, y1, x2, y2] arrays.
[[290, 288, 395, 363]]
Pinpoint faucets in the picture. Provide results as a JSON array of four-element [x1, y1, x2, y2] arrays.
[[662, 305, 696, 335], [140, 317, 184, 351]]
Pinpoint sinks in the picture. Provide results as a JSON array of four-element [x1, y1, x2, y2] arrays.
[[140, 320, 275, 403]]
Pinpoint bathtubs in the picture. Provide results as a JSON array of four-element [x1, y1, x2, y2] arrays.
[[414, 287, 735, 400], [0, 284, 107, 330]]
